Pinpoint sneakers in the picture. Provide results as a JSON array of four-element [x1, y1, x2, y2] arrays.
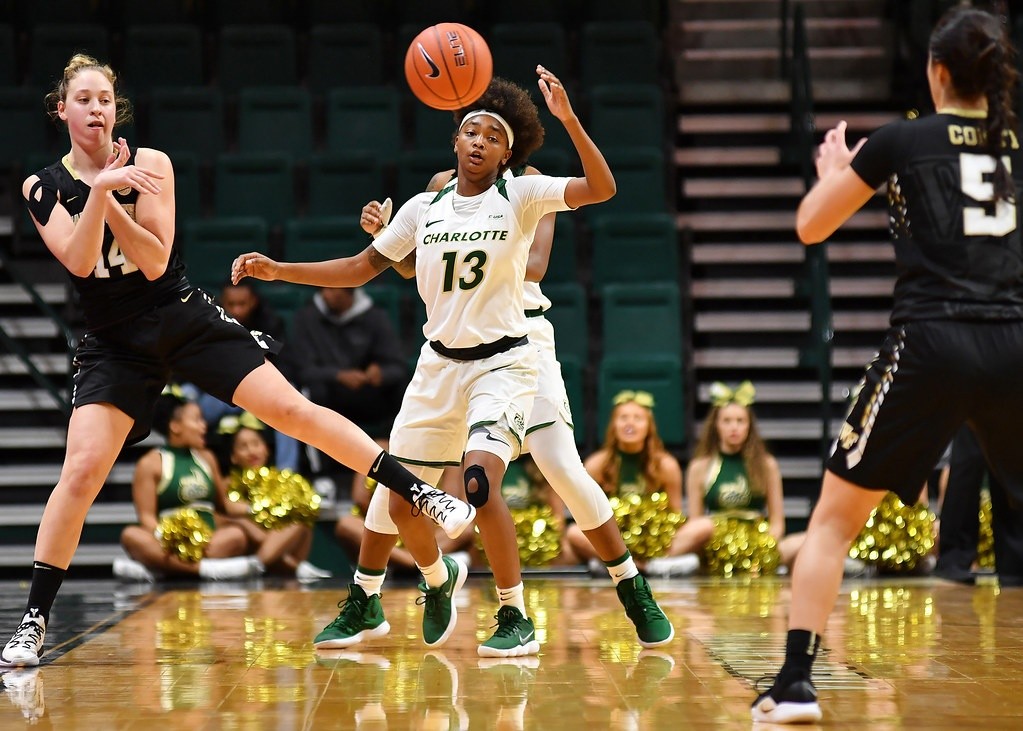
[[617, 651, 674, 709], [2, 669, 45, 716], [406, 482, 477, 539], [415, 555, 468, 647], [314, 650, 388, 708], [313, 583, 390, 648], [477, 605, 540, 657], [615, 569, 675, 648], [751, 674, 823, 723], [422, 652, 469, 731], [0, 606, 45, 667], [478, 657, 539, 704]]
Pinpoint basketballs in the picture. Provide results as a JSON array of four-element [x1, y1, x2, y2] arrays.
[[403, 22, 493, 110]]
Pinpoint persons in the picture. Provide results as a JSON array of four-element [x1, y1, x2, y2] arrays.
[[214, 425, 332, 579], [0, 53, 477, 668], [193, 279, 414, 495], [313, 166, 676, 650], [686, 381, 809, 576], [501, 391, 714, 577], [230, 65, 617, 657], [112, 394, 265, 584], [337, 439, 475, 575], [749, 11, 1023, 723], [842, 463, 992, 579], [0, 647, 675, 731]]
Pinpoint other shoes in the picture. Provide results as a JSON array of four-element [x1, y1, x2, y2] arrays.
[[997, 572, 1023, 586], [588, 558, 610, 578], [918, 555, 938, 576], [644, 552, 700, 577], [936, 565, 975, 584], [113, 558, 156, 583], [199, 556, 263, 580], [844, 558, 879, 577], [295, 562, 332, 580]]
